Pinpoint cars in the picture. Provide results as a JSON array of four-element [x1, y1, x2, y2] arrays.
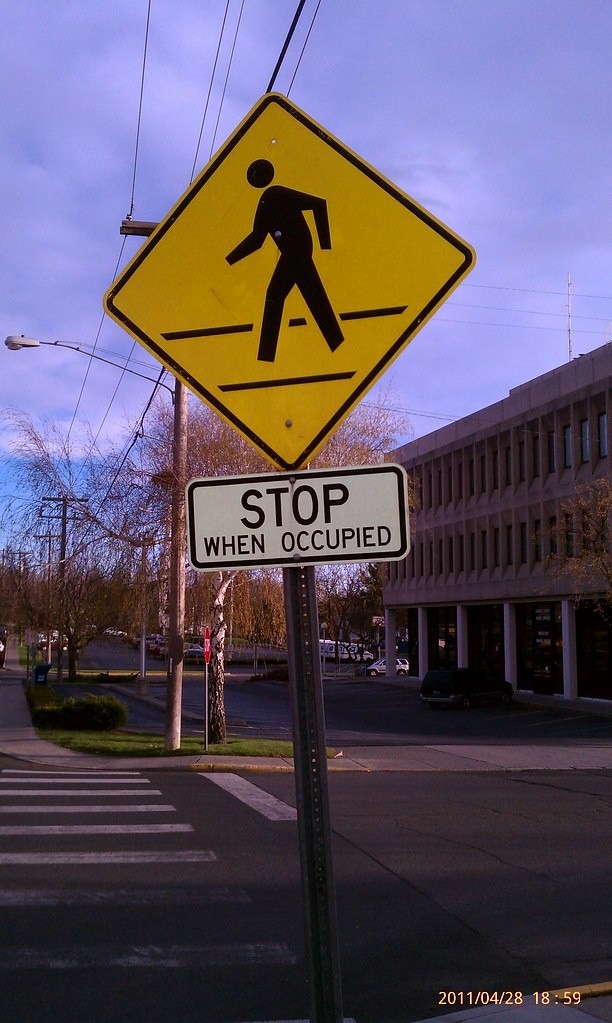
[[367, 658, 409, 677], [103, 626, 204, 658], [0, 627, 8, 667], [36, 629, 68, 651]]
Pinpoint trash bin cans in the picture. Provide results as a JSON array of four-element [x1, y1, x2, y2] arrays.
[[34, 663, 52, 685], [532, 668, 554, 696]]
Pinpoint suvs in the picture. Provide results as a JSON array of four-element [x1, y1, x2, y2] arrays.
[[419, 668, 514, 709]]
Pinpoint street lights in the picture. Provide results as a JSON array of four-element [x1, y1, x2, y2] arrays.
[[321, 622, 327, 673], [5, 334, 188, 750]]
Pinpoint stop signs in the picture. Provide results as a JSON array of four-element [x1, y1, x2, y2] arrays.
[[204, 627, 210, 663]]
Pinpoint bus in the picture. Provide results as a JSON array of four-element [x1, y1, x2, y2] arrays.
[[320, 640, 373, 662]]
